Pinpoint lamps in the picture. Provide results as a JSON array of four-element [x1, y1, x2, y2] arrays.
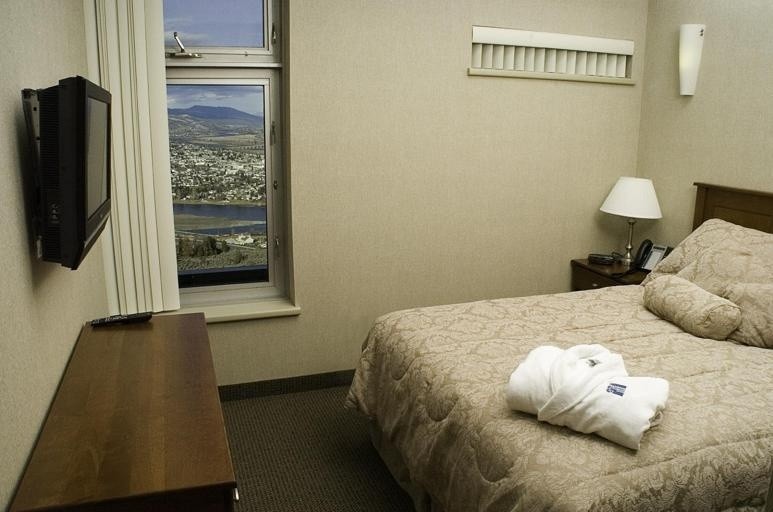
[[678, 23, 706, 96], [600, 177, 663, 267]]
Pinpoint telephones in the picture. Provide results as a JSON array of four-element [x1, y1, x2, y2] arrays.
[[633, 239, 674, 273]]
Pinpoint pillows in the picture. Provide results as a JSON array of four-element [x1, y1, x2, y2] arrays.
[[641, 219, 773, 349]]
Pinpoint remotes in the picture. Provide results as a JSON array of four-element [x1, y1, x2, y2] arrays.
[[90, 311, 155, 327]]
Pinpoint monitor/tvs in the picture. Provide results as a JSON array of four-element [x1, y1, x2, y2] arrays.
[[38, 75, 113, 271]]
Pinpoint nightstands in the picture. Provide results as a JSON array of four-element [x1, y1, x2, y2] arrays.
[[571, 259, 648, 290]]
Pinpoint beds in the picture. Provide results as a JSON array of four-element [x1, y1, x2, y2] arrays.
[[343, 182, 773, 512]]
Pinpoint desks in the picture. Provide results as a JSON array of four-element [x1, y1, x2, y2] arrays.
[[10, 313, 240, 511]]
